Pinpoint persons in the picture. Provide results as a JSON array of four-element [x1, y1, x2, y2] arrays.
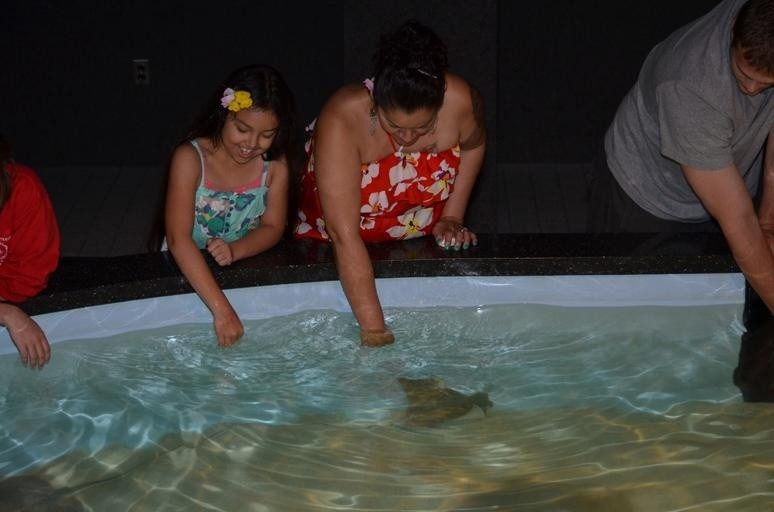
[[293, 59, 486, 347], [161, 83, 289, 348], [594, 0, 773, 311], [1, 155, 60, 370]]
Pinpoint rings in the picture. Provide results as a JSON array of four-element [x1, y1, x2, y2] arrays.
[[457, 224, 465, 232]]
